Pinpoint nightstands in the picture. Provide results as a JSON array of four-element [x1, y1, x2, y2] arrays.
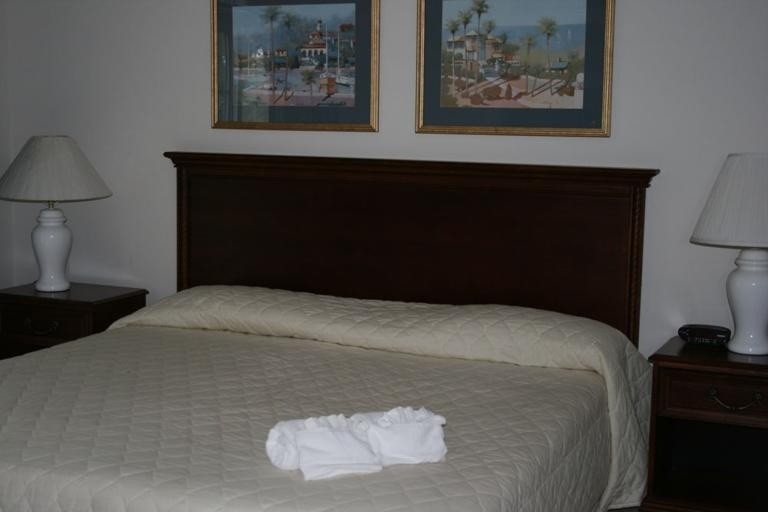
[[0, 280, 149, 362], [639, 336, 768, 512]]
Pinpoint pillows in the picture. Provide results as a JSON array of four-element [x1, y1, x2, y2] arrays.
[[107, 284, 631, 374]]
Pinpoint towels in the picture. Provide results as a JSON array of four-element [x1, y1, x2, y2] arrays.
[[264, 406, 448, 481]]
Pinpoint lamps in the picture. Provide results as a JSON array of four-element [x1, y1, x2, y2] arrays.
[[689, 153, 768, 357], [1, 136, 113, 292]]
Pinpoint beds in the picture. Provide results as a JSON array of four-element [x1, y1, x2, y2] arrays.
[[2, 152, 662, 511]]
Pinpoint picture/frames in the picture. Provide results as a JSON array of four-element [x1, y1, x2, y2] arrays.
[[209, 0, 381, 132], [414, 1, 616, 138]]
